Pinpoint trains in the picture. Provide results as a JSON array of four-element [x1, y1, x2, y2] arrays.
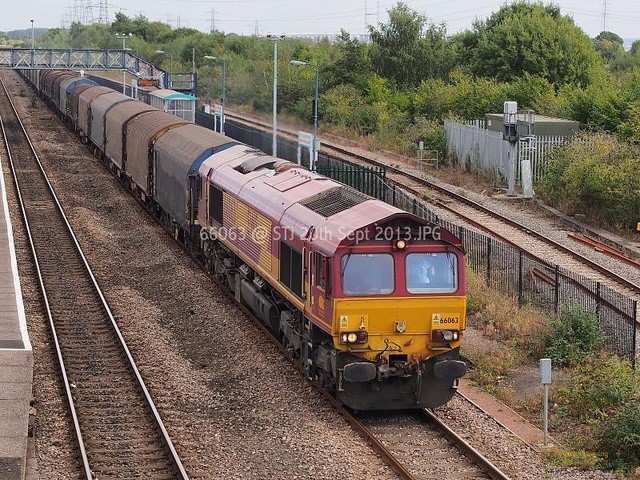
[[17, 69, 467, 414]]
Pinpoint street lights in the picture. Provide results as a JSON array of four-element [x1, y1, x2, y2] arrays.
[[122, 47, 138, 80], [202, 54, 227, 132], [156, 49, 172, 89], [288, 59, 321, 173]]
